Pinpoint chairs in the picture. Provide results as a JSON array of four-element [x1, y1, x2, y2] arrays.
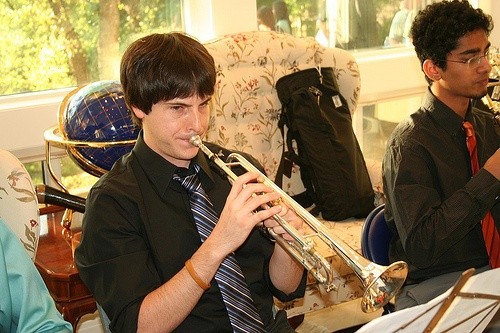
[[0, 149, 40, 260], [201, 31, 385, 319]]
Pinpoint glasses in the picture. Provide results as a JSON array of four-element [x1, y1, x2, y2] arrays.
[[436, 51, 492, 68]]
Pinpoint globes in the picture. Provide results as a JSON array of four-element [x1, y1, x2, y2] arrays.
[[44, 80, 142, 240]]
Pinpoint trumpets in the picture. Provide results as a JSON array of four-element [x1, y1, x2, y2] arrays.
[[483, 61, 500, 121], [188, 134, 408, 313]]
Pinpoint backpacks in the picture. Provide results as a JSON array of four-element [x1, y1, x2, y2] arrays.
[[274, 67, 375, 221]]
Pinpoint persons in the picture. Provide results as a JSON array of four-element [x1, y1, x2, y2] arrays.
[[74, 32, 306, 333], [382, 0, 500, 269], [0, 218, 73, 333]]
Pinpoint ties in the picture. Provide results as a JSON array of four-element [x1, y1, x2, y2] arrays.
[[462, 121, 500, 269], [171, 164, 267, 333]]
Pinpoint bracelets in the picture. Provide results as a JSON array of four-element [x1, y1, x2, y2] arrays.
[[185, 260, 212, 290]]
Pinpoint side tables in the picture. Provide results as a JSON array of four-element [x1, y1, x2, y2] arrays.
[[34, 228, 96, 325]]
